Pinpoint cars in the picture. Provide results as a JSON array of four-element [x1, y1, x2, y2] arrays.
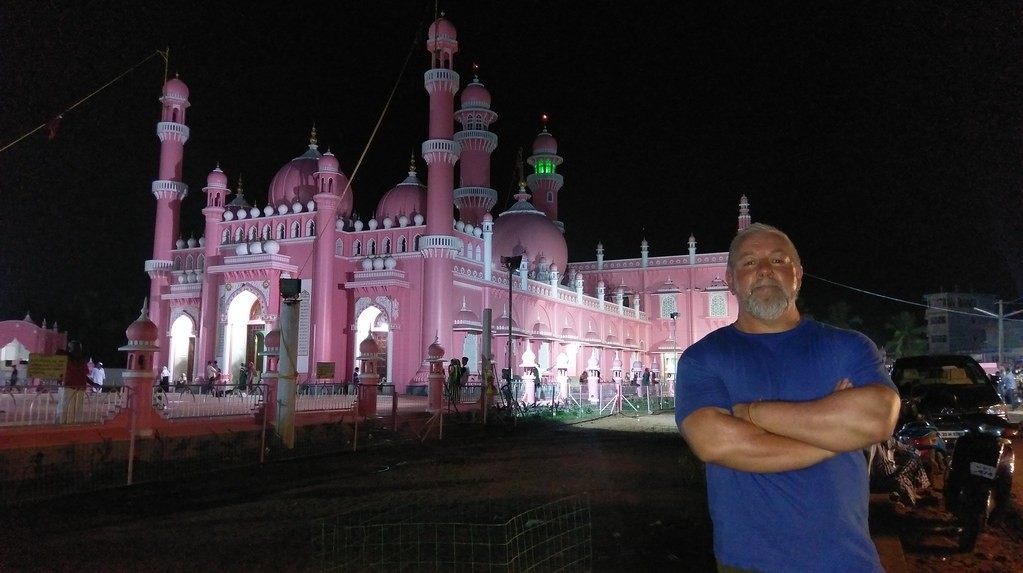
[[886, 355, 1010, 450]]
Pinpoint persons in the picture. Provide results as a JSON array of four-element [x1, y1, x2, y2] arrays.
[[991, 367, 1020, 410], [631, 368, 657, 395], [206, 360, 224, 395], [444, 356, 470, 403], [529, 366, 540, 393], [246, 361, 257, 394], [89, 361, 106, 395], [178, 373, 187, 384], [675, 224, 901, 573], [159, 365, 171, 391], [579, 370, 601, 384], [353, 367, 360, 394], [237, 363, 248, 393]]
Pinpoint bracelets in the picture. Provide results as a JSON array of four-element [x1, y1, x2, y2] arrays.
[[747, 401, 758, 427]]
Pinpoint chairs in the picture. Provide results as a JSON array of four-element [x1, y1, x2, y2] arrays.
[[945, 368, 974, 385], [899, 368, 922, 385]]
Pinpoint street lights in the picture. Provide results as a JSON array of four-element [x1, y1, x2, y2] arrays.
[[670, 312, 680, 380], [501, 256, 524, 416]]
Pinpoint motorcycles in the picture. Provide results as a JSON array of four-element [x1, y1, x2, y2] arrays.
[[937, 411, 1023, 553], [894, 393, 949, 480]]
